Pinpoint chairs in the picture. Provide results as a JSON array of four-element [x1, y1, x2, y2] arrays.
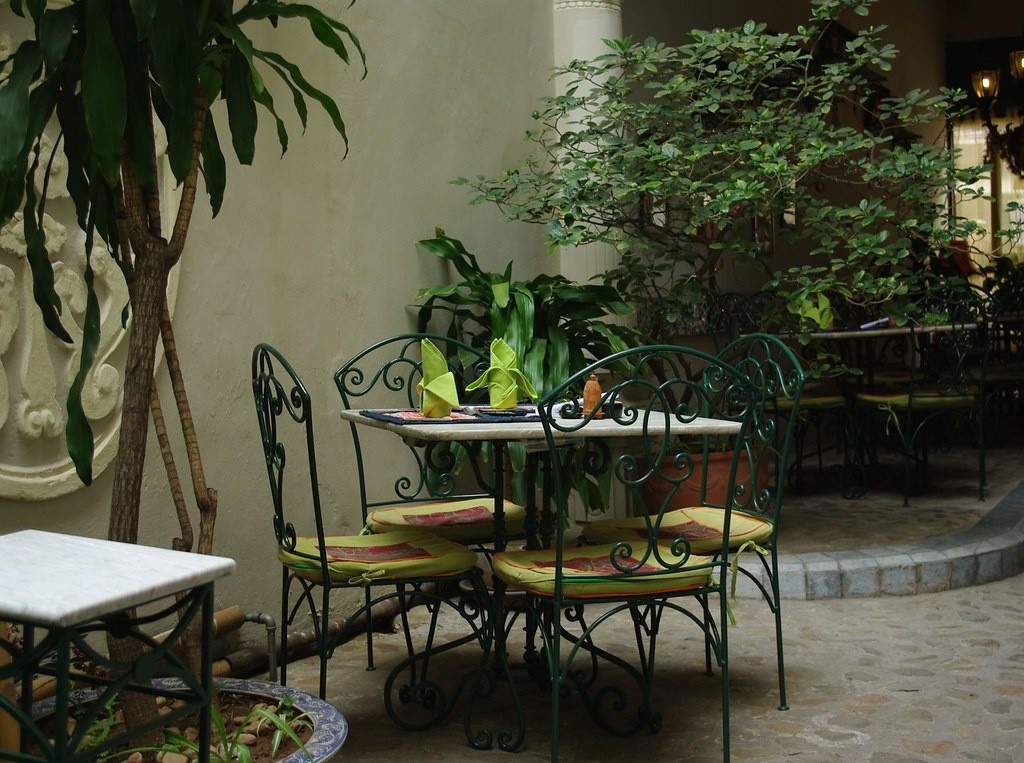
[[707, 283, 1023, 503], [250, 334, 790, 763]]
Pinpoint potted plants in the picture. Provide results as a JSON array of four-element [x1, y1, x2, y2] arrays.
[[0, 0, 367, 763], [407, 227, 666, 606], [450, 0, 1024, 511]]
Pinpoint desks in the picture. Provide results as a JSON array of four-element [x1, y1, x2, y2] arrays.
[[341, 401, 743, 752], [0, 528, 236, 762], [741, 324, 1004, 339]]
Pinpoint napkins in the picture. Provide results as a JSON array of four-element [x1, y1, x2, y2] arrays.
[[465, 339, 538, 407], [417, 338, 463, 418]]
